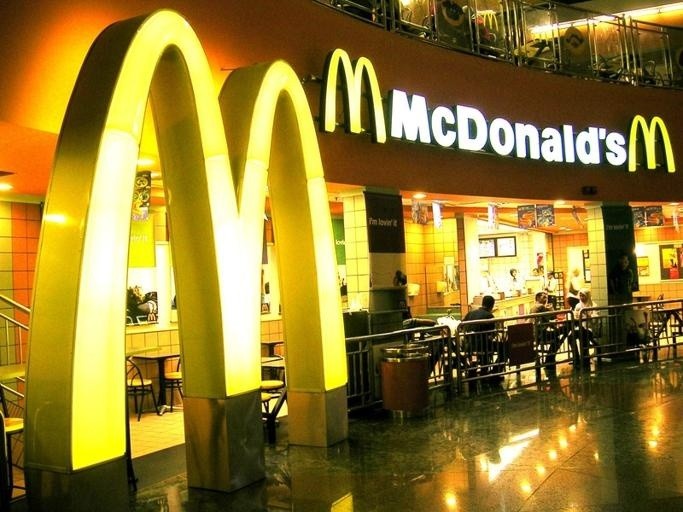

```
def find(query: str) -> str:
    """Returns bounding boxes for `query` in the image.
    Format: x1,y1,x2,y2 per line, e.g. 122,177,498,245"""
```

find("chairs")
1,388,27,512
377,304,504,394
163,358,183,414
649,308,681,338
127,357,159,421
256,340,294,445
351,0,683,91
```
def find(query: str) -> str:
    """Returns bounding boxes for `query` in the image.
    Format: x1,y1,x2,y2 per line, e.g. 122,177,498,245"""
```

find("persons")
482,266,558,310
609,250,634,303
567,287,601,370
457,294,509,384
566,264,584,313
525,290,563,370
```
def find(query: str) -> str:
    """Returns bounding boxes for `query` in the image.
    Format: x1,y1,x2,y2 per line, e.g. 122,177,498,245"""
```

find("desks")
134,347,184,415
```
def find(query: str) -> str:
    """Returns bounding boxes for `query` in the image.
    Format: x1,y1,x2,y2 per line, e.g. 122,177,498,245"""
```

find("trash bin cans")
381,344,431,418
